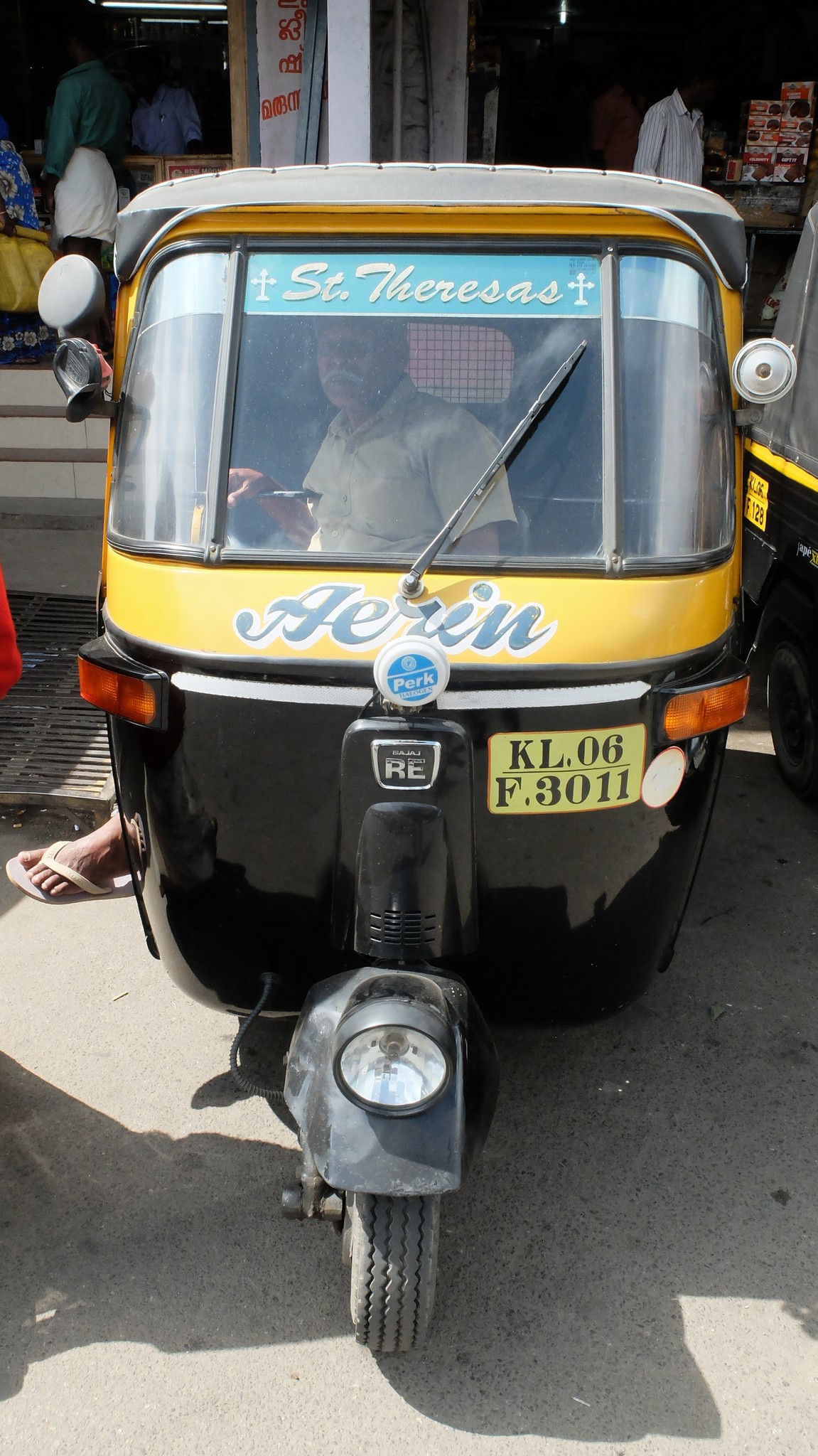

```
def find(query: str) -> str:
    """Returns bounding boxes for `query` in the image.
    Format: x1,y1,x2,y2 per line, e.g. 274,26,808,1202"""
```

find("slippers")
5,841,145,905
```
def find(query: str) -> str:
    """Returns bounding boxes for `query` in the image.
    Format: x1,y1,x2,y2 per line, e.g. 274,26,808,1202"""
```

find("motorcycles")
738,201,818,804
41,159,797,1357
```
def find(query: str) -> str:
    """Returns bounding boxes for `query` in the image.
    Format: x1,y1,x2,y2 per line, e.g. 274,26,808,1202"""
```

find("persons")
128,65,205,159
629,58,721,187
0,118,58,365
0,316,521,904
36,21,126,360
580,56,643,172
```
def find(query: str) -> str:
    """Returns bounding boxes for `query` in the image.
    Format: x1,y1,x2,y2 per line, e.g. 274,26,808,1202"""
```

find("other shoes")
98,344,114,360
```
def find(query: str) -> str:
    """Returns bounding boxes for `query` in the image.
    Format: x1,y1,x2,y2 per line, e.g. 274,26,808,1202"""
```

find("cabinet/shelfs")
707,177,812,336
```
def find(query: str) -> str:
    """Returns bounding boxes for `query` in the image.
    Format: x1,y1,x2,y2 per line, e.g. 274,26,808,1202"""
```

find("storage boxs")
704,79,816,186
